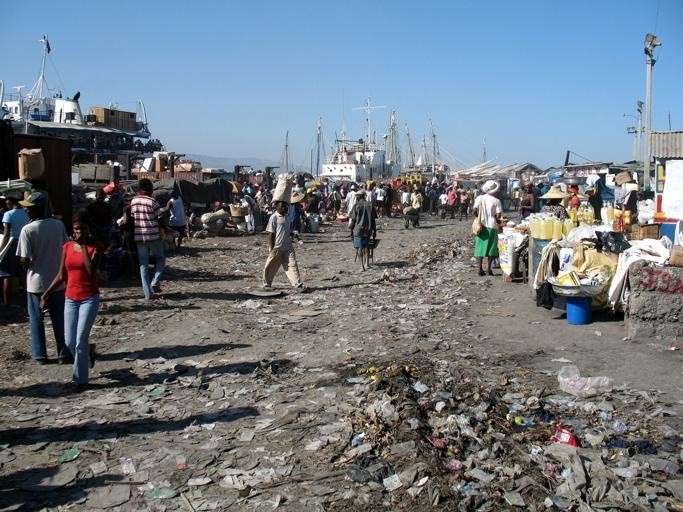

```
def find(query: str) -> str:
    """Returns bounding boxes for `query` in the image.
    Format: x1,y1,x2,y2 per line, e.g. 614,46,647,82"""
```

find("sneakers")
36,359,47,365
151,286,163,293
71,383,90,394
58,356,71,365
88,343,96,369
296,286,307,292
264,285,274,290
478,271,491,275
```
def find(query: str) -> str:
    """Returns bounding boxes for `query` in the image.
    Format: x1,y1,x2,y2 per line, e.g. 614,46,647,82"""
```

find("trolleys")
349,228,380,264
401,212,420,228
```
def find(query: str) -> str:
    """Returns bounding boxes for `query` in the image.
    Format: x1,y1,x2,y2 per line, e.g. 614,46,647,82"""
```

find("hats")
483,180,499,194
18,192,45,208
584,186,593,193
290,192,305,204
567,188,577,194
356,190,365,195
539,185,569,200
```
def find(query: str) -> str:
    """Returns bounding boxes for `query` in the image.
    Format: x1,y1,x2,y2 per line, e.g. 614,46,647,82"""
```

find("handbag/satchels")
472,217,482,235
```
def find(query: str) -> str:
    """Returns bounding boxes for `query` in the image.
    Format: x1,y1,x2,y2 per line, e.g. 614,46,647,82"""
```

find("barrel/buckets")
528,203,595,240
566,296,593,326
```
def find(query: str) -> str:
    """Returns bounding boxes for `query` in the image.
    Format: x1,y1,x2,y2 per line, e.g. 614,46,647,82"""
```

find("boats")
0,33,203,184
321,96,449,186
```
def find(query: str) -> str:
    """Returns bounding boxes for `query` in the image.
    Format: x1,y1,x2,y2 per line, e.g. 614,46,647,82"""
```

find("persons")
341,176,479,229
105,136,164,155
38,211,104,393
469,178,502,276
128,178,173,303
0,189,31,320
13,189,75,365
336,190,376,271
242,176,344,234
260,199,309,294
160,185,187,249
513,176,601,223
